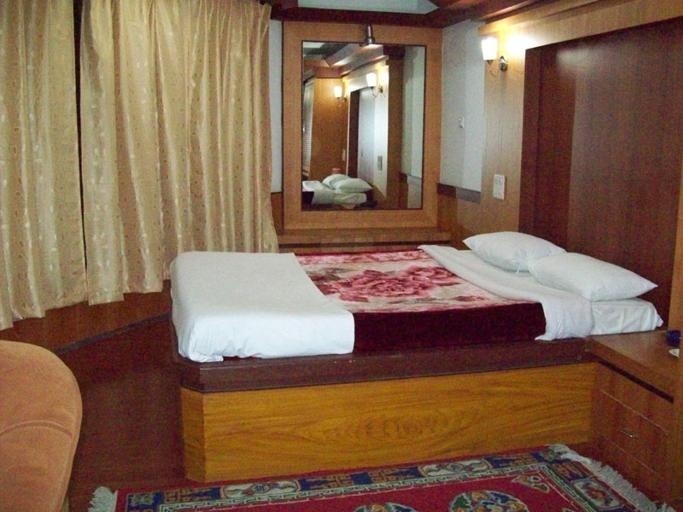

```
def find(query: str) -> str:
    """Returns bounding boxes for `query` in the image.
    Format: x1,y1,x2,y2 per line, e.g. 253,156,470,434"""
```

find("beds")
168,245,660,485
302,179,386,209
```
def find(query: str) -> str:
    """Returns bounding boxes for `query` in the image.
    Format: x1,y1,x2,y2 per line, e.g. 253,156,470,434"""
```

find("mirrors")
281,21,444,229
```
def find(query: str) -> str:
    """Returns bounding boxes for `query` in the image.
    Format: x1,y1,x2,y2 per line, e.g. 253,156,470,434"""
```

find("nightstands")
584,328,683,512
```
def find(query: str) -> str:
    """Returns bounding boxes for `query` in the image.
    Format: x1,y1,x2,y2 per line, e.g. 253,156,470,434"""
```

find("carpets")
83,442,676,511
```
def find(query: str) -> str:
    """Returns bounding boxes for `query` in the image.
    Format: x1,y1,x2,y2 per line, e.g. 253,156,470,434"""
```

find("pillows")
323,173,373,194
462,230,660,302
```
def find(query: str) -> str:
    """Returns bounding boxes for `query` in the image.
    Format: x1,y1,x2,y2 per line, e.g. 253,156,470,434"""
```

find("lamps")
362,24,383,50
363,70,389,98
479,36,509,71
333,85,348,102
